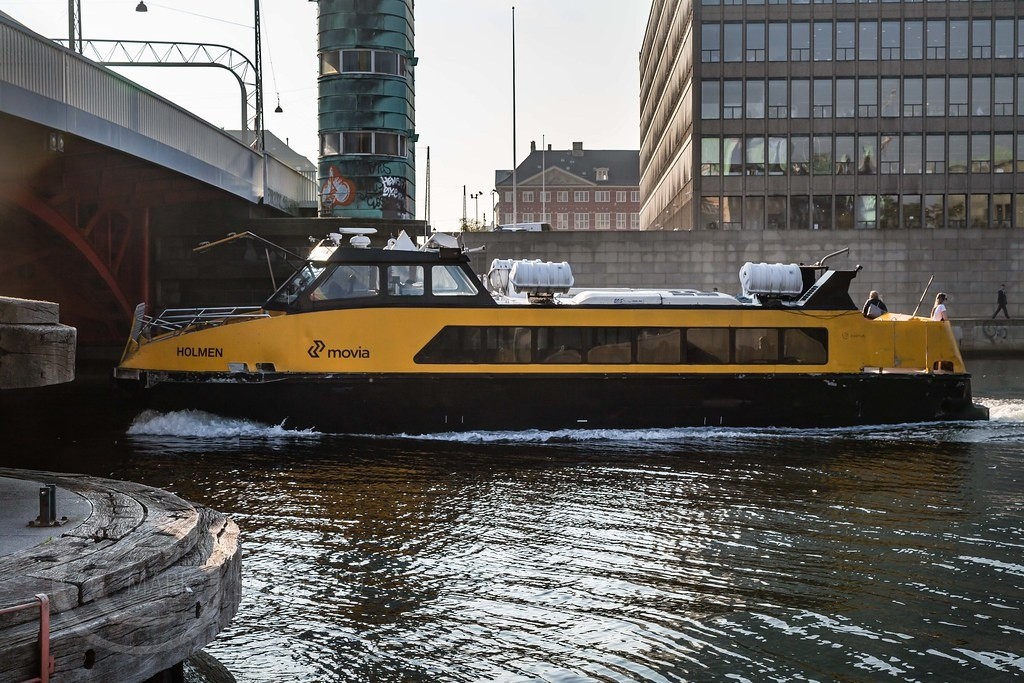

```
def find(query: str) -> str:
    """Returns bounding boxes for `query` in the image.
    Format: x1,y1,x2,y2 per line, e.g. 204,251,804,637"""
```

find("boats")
113,228,990,435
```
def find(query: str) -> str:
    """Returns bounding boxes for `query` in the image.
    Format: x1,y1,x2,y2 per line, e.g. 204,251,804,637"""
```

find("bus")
494,222,554,232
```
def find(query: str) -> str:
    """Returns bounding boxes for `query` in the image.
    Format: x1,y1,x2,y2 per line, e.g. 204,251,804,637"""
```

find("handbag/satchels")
867,302,883,318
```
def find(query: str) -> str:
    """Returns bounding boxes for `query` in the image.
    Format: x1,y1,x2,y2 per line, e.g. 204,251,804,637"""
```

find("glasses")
944,298,947,300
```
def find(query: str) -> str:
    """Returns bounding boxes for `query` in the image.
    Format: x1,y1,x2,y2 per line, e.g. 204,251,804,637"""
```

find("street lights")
471,191,483,228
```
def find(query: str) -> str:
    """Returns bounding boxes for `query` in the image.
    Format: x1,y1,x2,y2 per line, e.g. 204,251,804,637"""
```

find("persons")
930,293,948,321
992,284,1012,319
863,290,888,318
